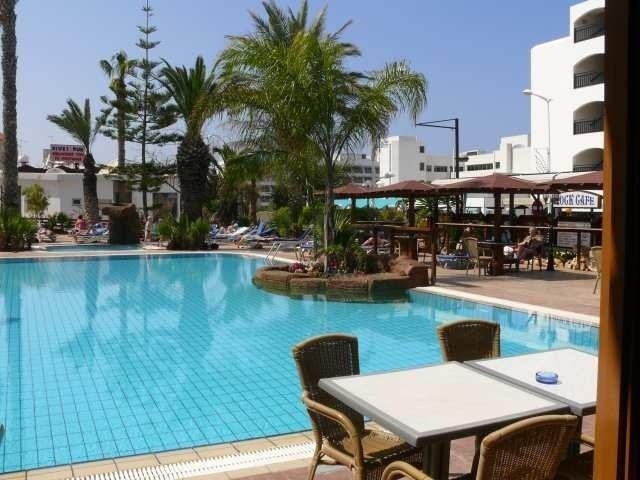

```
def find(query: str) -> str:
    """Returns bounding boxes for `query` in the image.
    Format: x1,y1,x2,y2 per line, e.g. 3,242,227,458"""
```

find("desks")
478,241,517,274
318,361,571,480
463,347,599,455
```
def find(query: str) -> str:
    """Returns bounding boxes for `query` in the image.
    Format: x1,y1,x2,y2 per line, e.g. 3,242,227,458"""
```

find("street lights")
523,89,558,172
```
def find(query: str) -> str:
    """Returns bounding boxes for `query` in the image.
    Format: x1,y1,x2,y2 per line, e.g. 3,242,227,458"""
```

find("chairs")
293,333,423,480
523,235,544,273
71,223,312,250
437,320,500,455
591,246,602,294
381,414,580,480
559,431,595,480
463,237,495,278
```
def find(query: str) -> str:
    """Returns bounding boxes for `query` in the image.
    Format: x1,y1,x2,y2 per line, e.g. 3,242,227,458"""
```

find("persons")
79,222,109,236
517,225,543,259
360,236,390,246
152,217,163,234
219,223,238,234
38,223,55,242
75,214,88,230
144,215,153,242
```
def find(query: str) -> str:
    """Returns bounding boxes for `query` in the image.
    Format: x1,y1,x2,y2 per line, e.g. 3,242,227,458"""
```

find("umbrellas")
535,169,605,191
364,178,435,227
313,182,377,223
430,173,563,226
